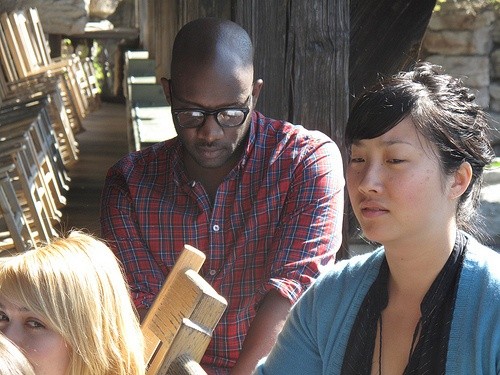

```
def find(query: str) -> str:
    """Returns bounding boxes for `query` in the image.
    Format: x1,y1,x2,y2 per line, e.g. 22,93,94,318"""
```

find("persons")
0,232,145,375
251,61,500,375
101,17,346,375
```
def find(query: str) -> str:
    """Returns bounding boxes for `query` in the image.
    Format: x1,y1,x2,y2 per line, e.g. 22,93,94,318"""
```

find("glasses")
168,79,256,129
